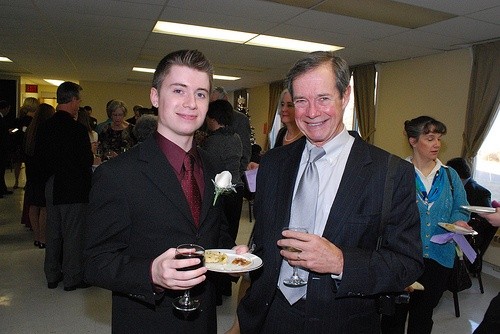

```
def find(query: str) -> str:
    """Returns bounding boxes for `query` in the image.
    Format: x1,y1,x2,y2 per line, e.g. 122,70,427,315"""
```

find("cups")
94,148,103,159
121,148,130,153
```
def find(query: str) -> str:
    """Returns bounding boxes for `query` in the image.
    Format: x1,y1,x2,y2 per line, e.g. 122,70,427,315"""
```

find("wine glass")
105,150,113,161
172,244,205,311
280,227,308,286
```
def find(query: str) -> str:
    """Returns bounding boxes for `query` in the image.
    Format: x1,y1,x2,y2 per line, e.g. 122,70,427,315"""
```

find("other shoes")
39,243,46,248
34,240,40,246
64,281,92,291
46,272,63,288
3,191,13,195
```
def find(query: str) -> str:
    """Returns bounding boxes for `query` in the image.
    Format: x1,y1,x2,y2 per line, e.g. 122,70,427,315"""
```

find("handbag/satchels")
444,167,482,279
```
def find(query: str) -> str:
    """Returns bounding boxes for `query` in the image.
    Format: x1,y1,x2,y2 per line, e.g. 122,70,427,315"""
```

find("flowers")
212,170,236,205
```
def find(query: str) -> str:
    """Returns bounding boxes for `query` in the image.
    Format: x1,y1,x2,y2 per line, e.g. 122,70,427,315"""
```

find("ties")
181,153,202,232
278,147,325,306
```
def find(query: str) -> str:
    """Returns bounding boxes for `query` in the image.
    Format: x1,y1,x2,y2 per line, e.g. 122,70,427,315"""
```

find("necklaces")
285,130,301,141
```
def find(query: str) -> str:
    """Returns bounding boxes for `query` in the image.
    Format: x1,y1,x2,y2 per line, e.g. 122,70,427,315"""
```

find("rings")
298,252,301,261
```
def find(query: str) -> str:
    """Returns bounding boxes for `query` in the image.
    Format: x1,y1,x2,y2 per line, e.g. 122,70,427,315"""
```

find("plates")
412,282,424,290
461,206,496,214
196,249,264,273
438,223,478,235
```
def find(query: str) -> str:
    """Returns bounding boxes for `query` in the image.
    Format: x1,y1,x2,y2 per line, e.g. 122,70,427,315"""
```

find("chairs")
452,216,500,317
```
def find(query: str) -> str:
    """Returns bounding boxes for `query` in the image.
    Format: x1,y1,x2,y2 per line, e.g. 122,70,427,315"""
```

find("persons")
79,50,247,334
237,50,424,334
473,206,500,334
274,88,304,148
446,157,493,273
0,81,264,291
380,115,472,334
